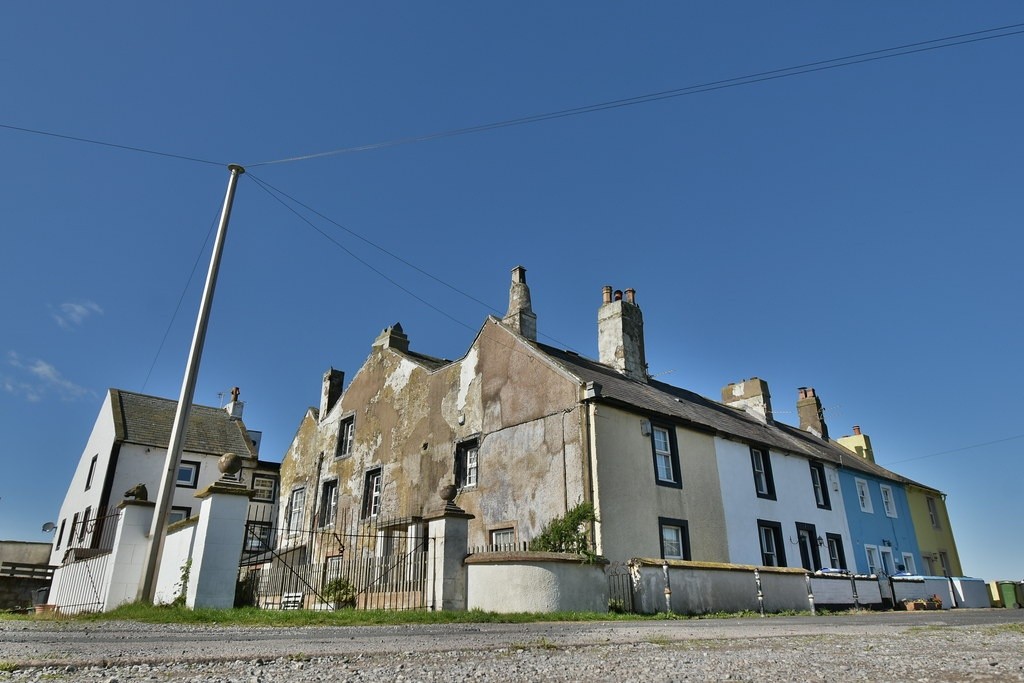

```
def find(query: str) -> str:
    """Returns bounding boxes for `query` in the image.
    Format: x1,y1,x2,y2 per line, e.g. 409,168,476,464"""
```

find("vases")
311,601,353,611
904,602,942,611
27,607,36,615
35,603,57,619
